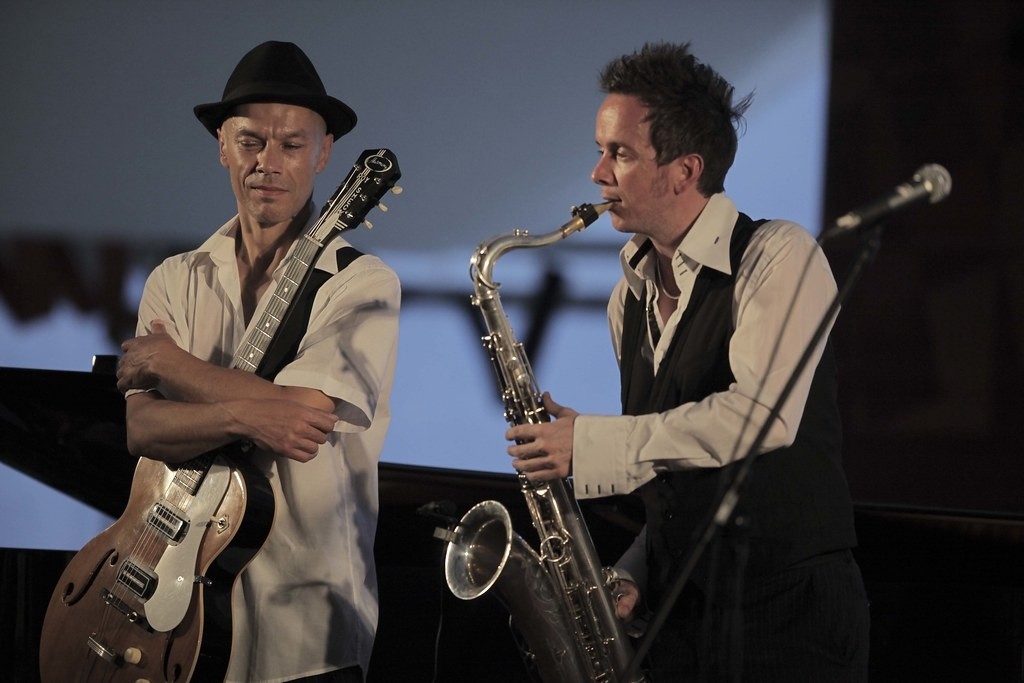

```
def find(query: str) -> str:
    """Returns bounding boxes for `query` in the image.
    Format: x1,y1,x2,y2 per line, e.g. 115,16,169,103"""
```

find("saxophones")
445,201,634,683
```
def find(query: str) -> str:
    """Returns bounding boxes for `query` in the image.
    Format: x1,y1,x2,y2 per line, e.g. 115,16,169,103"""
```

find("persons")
117,40,402,683
504,42,870,683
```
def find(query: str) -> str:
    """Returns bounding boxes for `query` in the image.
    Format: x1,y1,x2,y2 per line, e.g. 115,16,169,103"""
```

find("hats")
193,40,357,143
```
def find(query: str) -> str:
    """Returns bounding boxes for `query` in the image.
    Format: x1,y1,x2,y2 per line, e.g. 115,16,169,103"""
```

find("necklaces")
656,257,681,300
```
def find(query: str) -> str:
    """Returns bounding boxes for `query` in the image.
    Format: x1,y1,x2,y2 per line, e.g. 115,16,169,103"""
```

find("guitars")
38,148,402,683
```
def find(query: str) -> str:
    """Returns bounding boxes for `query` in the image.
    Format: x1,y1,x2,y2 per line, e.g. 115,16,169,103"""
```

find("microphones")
816,163,952,242
